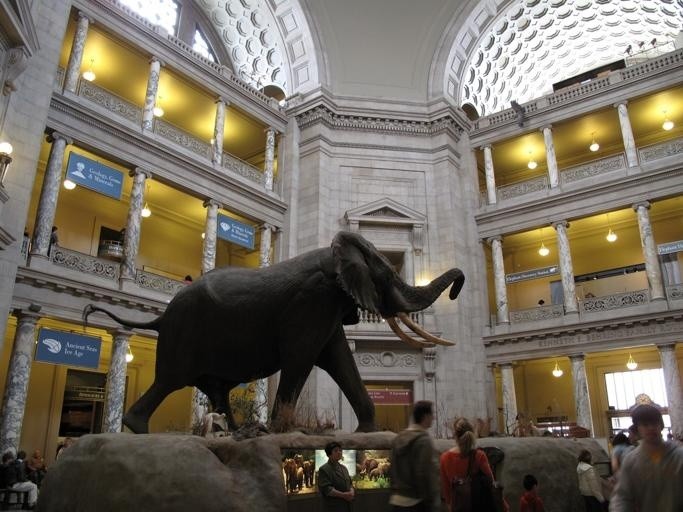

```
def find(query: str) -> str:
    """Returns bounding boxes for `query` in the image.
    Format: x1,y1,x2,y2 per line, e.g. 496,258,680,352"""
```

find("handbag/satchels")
451,449,504,512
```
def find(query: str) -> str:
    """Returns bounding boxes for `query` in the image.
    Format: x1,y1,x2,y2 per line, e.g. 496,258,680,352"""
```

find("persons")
517,473,547,511
27,448,47,485
316,441,356,511
583,291,596,298
447,418,468,439
0,450,13,487
182,274,193,287
536,298,546,307
45,223,60,258
439,417,509,511
55,436,73,461
387,399,436,511
4,449,39,508
575,402,683,511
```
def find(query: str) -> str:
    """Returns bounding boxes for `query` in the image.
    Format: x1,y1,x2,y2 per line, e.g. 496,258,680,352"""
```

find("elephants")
355,455,391,483
283,454,315,494
81,229,465,435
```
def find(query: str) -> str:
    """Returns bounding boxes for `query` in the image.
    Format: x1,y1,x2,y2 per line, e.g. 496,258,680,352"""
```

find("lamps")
140,181,153,217
83,59,96,82
525,148,538,170
151,92,167,118
585,129,603,152
548,355,565,378
603,212,617,244
535,226,551,258
623,349,638,371
658,108,674,130
